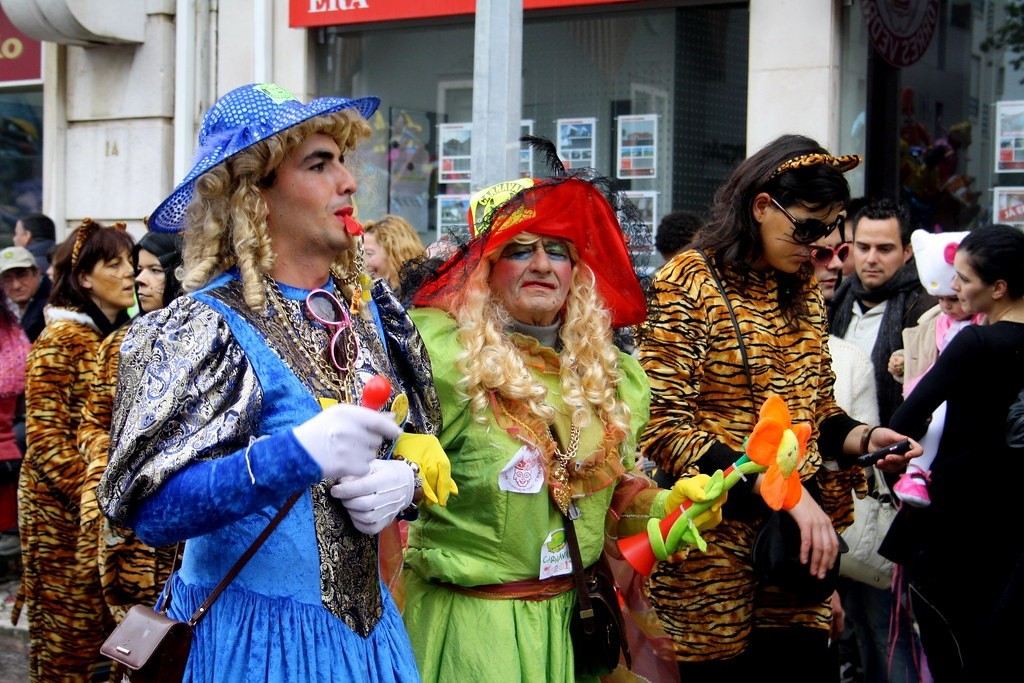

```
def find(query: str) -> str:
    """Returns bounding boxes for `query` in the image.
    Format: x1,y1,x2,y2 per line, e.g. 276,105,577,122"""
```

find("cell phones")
858,441,914,468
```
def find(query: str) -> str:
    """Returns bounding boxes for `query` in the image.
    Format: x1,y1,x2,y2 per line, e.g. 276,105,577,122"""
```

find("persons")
0,213,60,537
837,193,1024,683
79,229,188,683
394,177,650,683
98,83,413,683
16,213,136,683
632,133,925,683
360,215,424,294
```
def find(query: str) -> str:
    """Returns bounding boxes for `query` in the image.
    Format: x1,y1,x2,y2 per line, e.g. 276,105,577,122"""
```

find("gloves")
331,461,415,535
295,402,404,478
665,470,728,531
395,433,460,506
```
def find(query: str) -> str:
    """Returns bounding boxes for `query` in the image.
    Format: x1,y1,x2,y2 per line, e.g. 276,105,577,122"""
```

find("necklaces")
505,402,585,515
253,267,357,402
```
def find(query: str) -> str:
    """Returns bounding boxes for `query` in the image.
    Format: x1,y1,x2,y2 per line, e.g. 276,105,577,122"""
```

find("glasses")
771,198,837,245
307,289,358,370
811,243,850,269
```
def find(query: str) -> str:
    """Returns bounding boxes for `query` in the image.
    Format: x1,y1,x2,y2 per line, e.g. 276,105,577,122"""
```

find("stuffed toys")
647,393,812,563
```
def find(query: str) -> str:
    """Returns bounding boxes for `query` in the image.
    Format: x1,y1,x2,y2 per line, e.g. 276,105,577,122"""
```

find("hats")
401,135,648,331
911,228,971,296
149,84,381,236
0,246,39,274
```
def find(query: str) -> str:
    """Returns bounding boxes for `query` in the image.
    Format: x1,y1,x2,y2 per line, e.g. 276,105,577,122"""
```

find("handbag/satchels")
568,575,633,677
838,495,900,590
750,509,849,609
100,604,193,683
864,564,933,683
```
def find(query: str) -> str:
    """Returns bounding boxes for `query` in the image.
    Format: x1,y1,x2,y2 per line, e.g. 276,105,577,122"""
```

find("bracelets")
859,424,880,456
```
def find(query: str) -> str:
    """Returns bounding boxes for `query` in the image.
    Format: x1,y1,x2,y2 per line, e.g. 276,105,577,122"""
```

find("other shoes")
894,469,934,507
0,532,20,555
